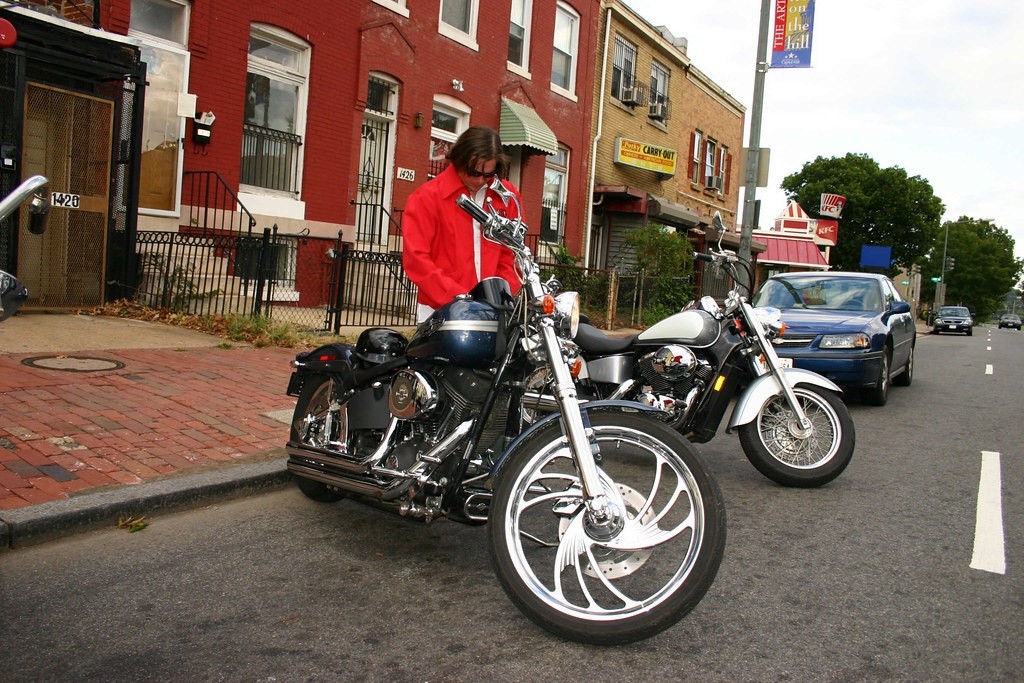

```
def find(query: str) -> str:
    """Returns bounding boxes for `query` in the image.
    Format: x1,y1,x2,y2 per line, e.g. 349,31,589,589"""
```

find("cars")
743,270,918,408
931,306,976,337
998,313,1024,331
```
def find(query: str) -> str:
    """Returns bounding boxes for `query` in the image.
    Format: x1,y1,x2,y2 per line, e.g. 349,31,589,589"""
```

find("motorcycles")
278,193,729,648
508,209,856,490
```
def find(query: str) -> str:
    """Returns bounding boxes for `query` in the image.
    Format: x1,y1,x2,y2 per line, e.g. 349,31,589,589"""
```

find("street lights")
938,218,995,306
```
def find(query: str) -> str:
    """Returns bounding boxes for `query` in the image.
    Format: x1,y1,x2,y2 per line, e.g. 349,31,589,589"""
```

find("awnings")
499,97,558,157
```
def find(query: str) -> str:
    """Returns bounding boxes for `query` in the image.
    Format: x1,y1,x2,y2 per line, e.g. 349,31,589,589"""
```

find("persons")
404,125,527,471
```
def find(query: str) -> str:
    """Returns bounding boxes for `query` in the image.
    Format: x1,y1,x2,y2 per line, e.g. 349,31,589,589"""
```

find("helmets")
353,328,408,364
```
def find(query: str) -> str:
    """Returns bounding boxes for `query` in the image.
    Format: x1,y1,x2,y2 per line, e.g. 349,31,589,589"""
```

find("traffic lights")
946,256,955,272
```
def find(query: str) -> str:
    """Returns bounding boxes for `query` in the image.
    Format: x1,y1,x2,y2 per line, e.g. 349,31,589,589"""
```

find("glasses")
467,167,496,178
